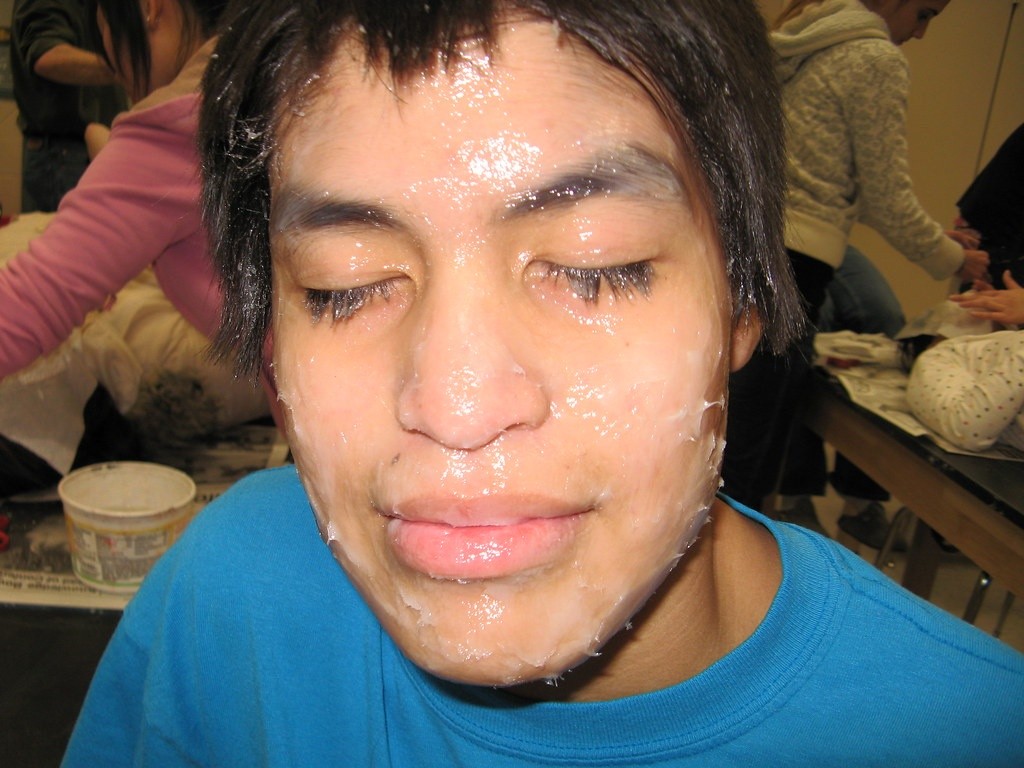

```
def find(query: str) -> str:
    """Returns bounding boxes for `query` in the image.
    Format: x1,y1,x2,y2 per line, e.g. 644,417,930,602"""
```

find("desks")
805,362,1024,642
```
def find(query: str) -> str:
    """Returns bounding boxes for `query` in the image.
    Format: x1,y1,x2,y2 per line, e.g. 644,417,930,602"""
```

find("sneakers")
772,498,827,540
836,502,906,551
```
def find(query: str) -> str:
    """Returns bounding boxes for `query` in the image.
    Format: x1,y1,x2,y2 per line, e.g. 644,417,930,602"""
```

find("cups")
58,460,197,593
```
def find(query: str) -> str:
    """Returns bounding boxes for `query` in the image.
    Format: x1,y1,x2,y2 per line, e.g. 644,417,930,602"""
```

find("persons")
715,0,1024,558
59,0,1024,768
0,0,294,463
